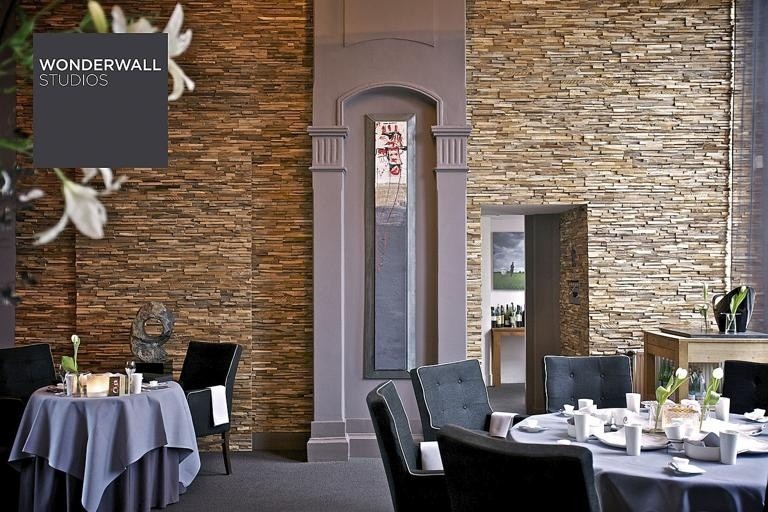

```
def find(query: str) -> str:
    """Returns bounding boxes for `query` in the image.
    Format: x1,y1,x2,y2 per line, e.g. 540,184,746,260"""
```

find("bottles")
491,302,525,328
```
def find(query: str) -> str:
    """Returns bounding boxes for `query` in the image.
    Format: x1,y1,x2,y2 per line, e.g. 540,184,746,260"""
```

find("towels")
420,442,443,471
206,384,229,427
488,411,519,439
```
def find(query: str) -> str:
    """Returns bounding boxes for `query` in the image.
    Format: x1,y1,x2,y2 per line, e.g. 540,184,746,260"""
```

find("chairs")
411,360,525,445
180,341,244,475
437,425,600,512
0,343,57,456
364,379,450,510
543,353,633,411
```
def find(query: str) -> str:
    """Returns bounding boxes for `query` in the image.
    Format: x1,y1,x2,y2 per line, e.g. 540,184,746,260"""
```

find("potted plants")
719,288,749,334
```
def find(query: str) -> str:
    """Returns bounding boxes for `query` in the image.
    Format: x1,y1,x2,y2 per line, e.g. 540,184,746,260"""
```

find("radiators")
617,349,723,401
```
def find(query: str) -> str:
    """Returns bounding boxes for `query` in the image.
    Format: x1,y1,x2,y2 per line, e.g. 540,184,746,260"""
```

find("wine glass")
58,364,71,397
125,361,136,381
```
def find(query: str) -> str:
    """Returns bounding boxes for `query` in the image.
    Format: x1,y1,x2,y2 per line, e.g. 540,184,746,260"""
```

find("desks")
491,328,525,386
644,328,768,409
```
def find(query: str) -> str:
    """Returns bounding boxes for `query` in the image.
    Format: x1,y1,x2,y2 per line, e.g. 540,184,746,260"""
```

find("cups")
150,381,158,387
132,373,142,395
527,393,766,465
66,374,77,395
